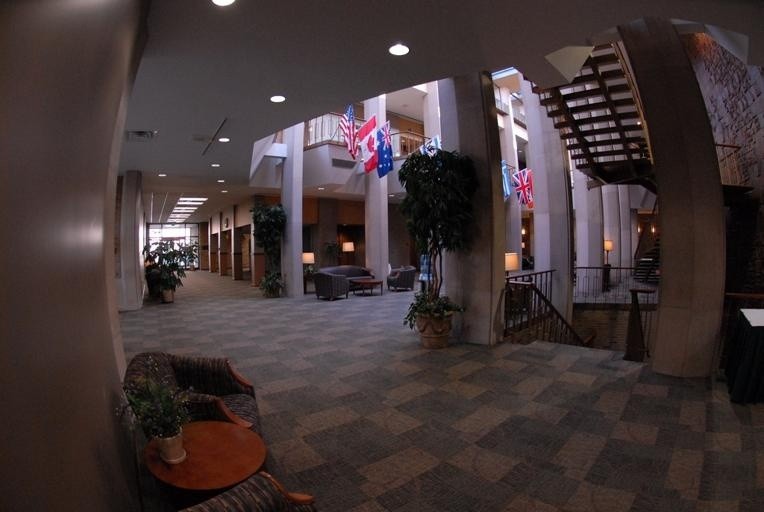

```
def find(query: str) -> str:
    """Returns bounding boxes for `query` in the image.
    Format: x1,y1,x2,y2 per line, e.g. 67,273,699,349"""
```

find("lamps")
504,252,519,277
342,241,356,265
602,239,614,266
302,252,315,265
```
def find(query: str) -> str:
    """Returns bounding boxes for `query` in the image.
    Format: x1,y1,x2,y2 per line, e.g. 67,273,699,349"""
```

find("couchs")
320,265,374,296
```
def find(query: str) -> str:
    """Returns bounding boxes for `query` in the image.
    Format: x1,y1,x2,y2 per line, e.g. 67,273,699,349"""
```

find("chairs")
171,469,317,512
313,271,349,303
118,345,267,438
386,266,416,292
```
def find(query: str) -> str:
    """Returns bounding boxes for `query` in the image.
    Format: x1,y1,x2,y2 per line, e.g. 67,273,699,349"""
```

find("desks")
137,418,269,490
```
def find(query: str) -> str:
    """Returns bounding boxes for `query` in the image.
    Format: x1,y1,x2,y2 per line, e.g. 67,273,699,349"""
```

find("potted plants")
394,143,482,348
243,201,289,299
138,237,200,305
110,350,193,465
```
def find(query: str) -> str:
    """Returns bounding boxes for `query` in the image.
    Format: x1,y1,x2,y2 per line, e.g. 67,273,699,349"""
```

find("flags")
375,122,394,179
513,168,535,206
502,160,513,202
419,135,440,158
355,113,377,174
338,103,359,161
